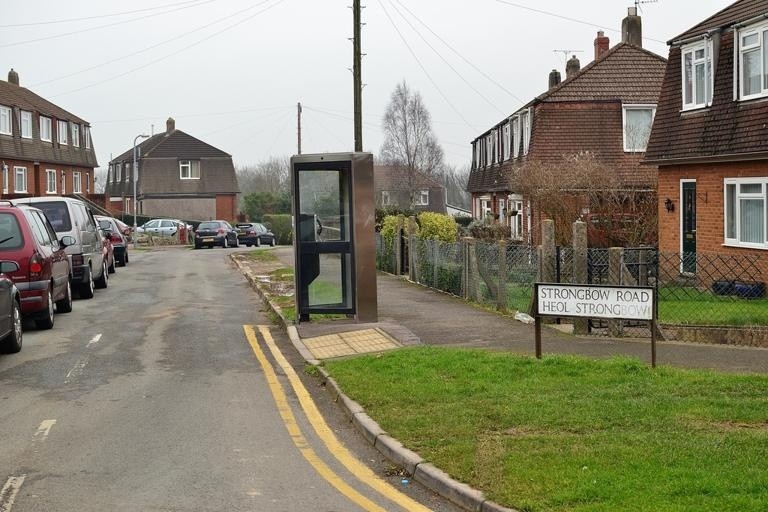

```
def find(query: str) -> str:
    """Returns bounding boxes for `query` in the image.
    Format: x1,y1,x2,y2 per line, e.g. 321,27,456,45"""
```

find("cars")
195,220,240,248
1,196,132,354
581,212,658,248
137,219,193,237
234,223,276,247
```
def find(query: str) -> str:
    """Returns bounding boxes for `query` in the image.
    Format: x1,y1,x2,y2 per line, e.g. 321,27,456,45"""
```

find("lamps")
503,208,506,215
665,198,674,213
61,171,66,177
93,177,97,182
1,161,5,169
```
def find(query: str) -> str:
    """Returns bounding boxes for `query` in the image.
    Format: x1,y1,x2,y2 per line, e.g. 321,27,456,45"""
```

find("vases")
712,280,736,295
734,280,765,299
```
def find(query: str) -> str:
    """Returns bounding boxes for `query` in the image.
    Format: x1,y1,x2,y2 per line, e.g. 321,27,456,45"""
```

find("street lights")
133,134,149,249
